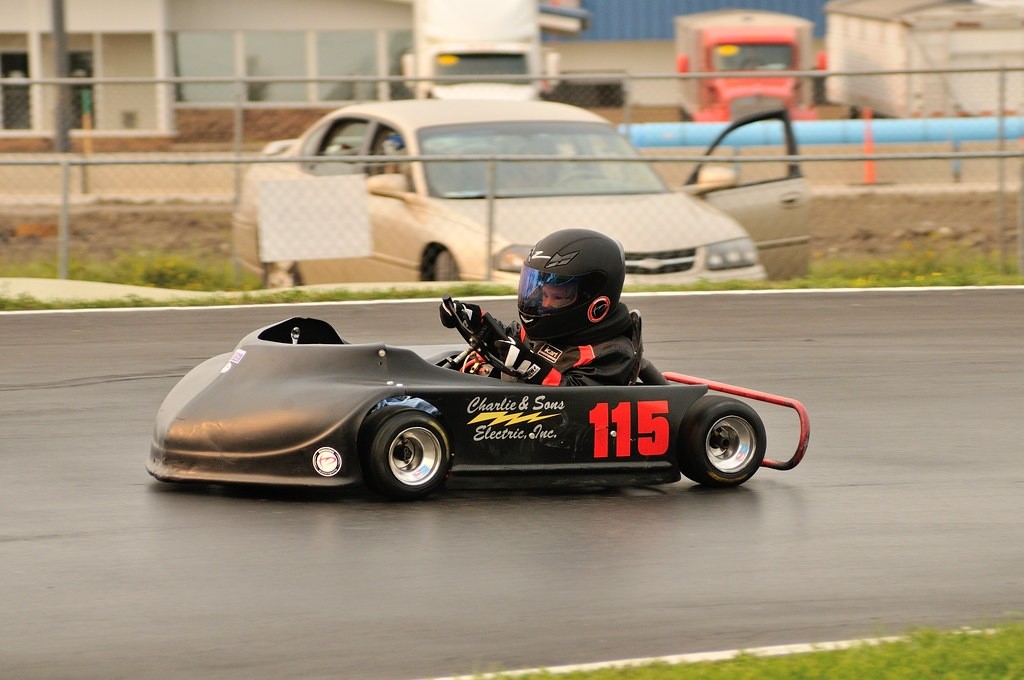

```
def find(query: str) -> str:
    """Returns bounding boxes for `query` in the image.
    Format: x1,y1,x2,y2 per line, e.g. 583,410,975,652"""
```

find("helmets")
517,229,625,337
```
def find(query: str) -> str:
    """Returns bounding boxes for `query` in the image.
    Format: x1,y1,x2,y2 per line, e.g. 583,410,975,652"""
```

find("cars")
230,98,813,291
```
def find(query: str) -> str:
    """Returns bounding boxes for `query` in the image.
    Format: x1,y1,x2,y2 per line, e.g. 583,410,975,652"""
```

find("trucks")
401,0,562,103
675,8,828,121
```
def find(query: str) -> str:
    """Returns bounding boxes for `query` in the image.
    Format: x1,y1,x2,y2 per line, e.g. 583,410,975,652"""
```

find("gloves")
439,300,481,330
494,333,554,384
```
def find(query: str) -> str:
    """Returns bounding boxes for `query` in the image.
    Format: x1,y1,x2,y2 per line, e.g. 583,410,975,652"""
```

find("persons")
439,228,636,387
